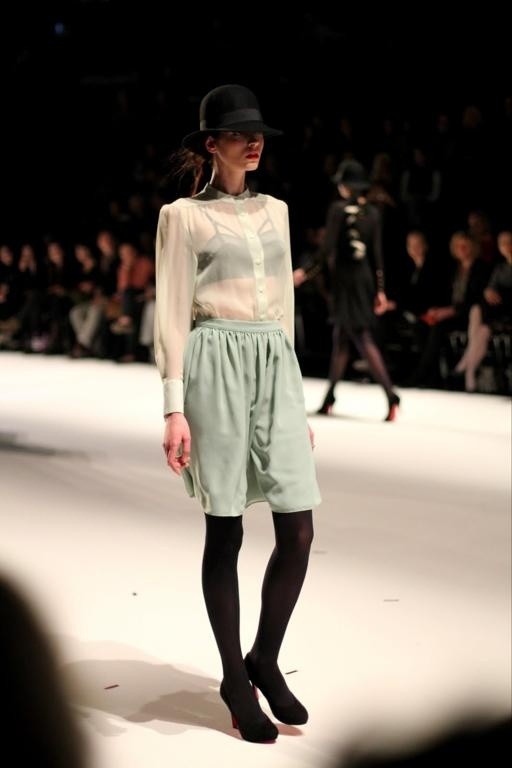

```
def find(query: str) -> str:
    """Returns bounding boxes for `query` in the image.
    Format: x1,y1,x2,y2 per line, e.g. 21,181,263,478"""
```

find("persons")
293,158,401,422
154,86,322,746
297,226,512,397
1,233,156,366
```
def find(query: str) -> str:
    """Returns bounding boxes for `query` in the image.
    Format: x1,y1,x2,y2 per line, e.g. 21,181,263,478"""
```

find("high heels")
219,674,278,743
242,650,312,725
383,394,400,423
316,392,335,416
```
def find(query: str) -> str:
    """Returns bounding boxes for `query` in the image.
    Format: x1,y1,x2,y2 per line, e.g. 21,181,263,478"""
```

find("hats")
328,159,373,193
182,83,285,160
